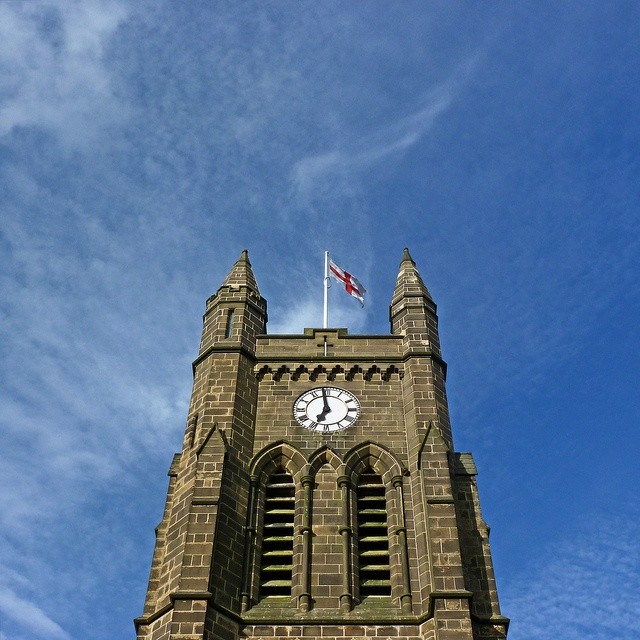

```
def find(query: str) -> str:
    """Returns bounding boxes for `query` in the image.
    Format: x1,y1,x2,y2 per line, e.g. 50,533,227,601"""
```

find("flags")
323,251,367,307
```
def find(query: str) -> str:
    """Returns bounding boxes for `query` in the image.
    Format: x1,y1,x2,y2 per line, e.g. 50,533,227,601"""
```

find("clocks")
291,384,363,435
